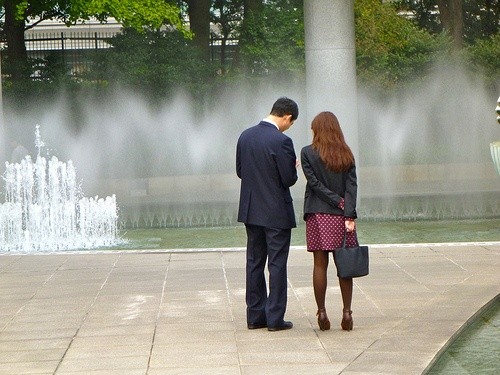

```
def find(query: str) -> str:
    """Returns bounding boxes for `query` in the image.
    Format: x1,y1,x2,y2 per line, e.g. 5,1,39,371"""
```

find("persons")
11,137,29,163
236,98,300,331
300,112,360,331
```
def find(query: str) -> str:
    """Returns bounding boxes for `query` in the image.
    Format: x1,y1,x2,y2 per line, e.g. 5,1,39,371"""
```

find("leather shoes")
248,322,267,329
268,321,293,331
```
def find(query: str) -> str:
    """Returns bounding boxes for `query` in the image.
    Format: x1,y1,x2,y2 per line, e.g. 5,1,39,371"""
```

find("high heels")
341,311,353,332
316,310,330,331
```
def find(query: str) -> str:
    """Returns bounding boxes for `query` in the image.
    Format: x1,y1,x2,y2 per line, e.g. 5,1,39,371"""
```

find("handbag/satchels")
334,228,369,279
